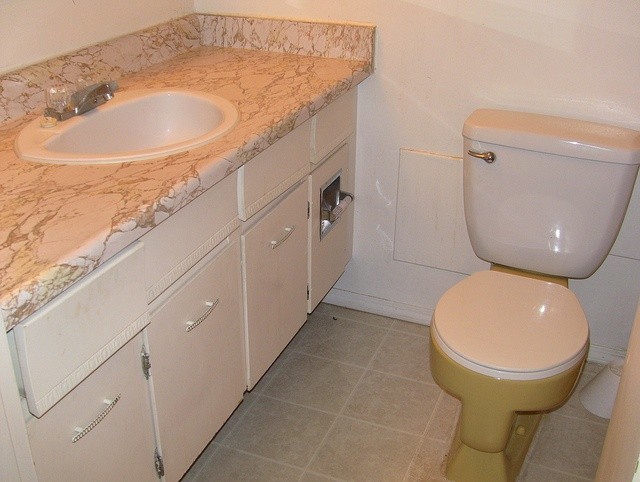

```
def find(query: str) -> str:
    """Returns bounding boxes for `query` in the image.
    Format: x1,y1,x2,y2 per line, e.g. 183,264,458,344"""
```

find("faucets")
68,80,118,115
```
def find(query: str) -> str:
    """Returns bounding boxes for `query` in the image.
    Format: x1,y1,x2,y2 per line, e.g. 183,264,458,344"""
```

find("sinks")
40,89,228,156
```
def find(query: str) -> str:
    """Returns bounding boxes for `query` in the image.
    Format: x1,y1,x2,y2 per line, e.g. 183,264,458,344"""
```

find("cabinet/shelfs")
308,84,359,314
7,238,166,481
137,167,247,482
236,116,315,394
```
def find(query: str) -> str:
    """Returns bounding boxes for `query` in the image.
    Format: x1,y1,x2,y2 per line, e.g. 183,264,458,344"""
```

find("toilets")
428,106,640,482
